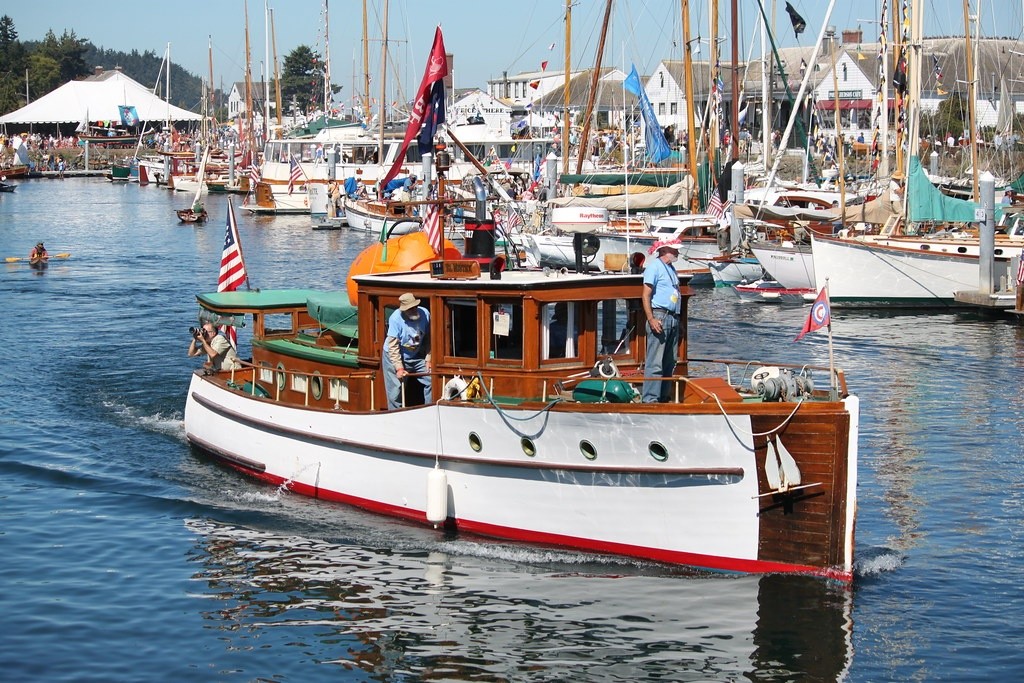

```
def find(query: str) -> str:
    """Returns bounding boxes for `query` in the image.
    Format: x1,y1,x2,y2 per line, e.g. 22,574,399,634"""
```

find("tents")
0,70,221,124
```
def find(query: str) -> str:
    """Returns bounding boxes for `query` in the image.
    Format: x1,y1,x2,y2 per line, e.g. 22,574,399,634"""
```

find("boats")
184,23,859,583
178,144,210,223
29,257,48,270
0,167,30,192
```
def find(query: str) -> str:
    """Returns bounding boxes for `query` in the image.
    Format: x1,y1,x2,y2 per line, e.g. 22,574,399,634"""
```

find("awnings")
816,99,903,110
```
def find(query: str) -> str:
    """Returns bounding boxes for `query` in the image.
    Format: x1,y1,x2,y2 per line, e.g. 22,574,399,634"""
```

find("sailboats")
106,0,1024,326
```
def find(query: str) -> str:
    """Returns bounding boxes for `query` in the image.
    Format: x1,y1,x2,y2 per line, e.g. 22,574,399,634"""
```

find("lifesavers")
443,378,468,400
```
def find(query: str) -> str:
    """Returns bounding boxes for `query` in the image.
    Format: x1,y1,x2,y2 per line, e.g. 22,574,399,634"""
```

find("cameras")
189,326,207,338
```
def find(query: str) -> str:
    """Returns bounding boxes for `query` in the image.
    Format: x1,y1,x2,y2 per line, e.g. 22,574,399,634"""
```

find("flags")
380,29,447,194
251,155,261,183
785,1,806,36
288,156,302,194
216,204,245,351
424,186,441,253
707,188,731,219
621,65,670,164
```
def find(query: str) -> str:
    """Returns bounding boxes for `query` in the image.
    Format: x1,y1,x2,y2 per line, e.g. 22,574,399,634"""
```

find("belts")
652,308,678,317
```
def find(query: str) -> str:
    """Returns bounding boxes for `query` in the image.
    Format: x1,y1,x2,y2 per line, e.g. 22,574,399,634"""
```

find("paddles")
5,253,70,262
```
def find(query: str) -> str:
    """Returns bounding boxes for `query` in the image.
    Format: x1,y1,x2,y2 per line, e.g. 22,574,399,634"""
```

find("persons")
194,200,203,213
188,323,241,370
29,241,49,261
642,235,685,403
2,131,98,171
328,148,379,217
918,131,1020,153
890,170,905,235
144,125,239,153
383,293,432,410
236,163,243,186
664,124,782,156
402,175,418,215
1003,186,1014,204
549,128,642,156
807,132,865,157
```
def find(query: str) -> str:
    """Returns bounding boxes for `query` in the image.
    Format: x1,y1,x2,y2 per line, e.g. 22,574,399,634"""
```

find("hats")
398,292,421,310
654,234,684,251
